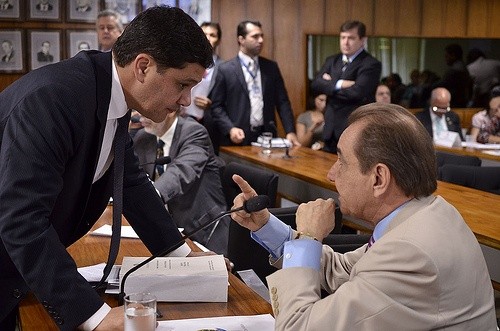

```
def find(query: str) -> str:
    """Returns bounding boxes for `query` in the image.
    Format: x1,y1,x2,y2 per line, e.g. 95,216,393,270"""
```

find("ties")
95,108,132,290
248,59,263,122
363,235,375,253
435,117,445,136
157,140,166,176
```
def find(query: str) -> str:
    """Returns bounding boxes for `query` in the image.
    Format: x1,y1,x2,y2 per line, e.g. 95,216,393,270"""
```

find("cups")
261,133,272,155
123,292,157,331
466,135,475,152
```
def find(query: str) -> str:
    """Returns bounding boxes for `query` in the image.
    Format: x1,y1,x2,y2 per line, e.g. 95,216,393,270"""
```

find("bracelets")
294,232,318,241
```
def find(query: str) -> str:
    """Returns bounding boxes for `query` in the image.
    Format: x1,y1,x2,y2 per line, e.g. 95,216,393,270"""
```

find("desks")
433,142,500,162
17,202,276,331
220,142,500,291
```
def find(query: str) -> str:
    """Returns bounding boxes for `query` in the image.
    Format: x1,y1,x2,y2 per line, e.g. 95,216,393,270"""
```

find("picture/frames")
0,0,212,76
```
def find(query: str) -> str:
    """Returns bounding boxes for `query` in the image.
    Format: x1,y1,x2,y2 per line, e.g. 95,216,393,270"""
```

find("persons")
210,20,302,147
77,41,90,51
232,102,498,331
133,107,231,258
310,20,382,154
1,40,18,65
96,12,123,52
465,50,500,107
375,69,440,109
181,21,223,157
0,5,216,331
416,86,465,143
295,89,327,149
0,0,13,10
440,43,474,109
36,0,53,11
75,1,204,17
37,39,53,62
470,89,500,144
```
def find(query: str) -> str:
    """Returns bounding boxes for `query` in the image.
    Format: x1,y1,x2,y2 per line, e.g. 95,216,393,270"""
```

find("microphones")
270,120,293,159
119,195,270,307
141,155,172,167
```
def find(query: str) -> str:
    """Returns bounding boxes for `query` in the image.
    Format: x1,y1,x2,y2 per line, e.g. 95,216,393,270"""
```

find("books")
119,254,227,302
